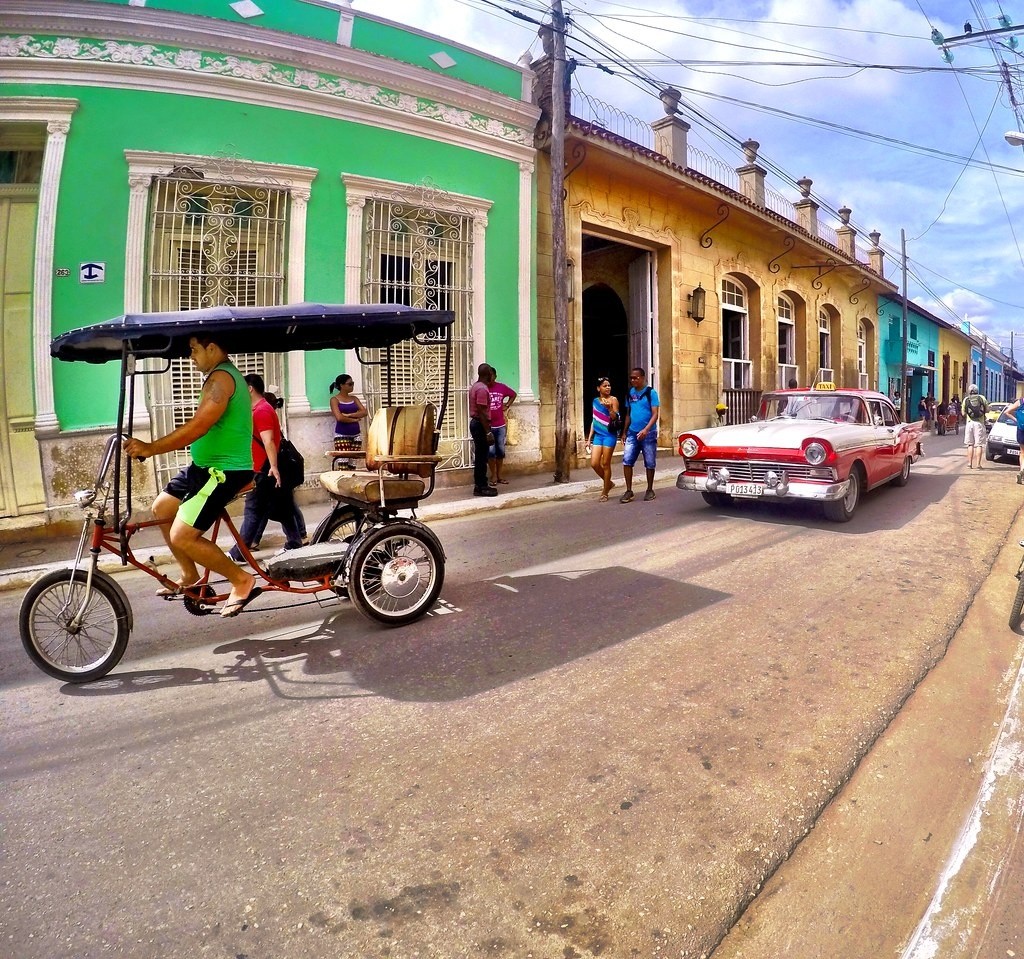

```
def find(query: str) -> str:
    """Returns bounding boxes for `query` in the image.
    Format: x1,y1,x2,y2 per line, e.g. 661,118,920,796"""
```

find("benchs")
319,401,435,510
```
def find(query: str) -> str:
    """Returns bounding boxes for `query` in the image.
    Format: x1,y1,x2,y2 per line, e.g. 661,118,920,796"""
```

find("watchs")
505,404,509,408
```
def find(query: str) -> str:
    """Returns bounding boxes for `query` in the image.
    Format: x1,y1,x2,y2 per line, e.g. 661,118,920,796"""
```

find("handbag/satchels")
262,436,304,487
608,411,622,437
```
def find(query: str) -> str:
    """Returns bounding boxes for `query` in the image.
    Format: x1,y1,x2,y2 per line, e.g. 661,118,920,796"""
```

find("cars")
676,382,925,522
985,403,1021,465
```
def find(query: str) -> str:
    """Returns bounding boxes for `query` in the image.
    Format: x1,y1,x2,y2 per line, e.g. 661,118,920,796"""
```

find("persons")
876,391,901,418
225,373,308,564
584,378,619,502
123,330,255,616
962,385,990,470
777,379,797,415
469,364,498,496
620,368,660,502
1005,398,1024,483
919,392,962,431
330,374,368,471
489,368,517,486
707,403,728,427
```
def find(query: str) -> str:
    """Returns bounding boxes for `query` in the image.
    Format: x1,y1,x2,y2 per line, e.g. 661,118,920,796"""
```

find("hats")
968,384,978,394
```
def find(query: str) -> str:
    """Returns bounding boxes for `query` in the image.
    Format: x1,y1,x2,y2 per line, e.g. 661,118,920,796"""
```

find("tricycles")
18,304,455,682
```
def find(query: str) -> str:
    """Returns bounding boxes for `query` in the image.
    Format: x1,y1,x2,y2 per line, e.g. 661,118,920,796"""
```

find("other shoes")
603,483,615,495
473,486,498,497
600,495,608,502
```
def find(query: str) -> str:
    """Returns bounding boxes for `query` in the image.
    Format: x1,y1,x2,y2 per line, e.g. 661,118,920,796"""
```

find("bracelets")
487,431,491,435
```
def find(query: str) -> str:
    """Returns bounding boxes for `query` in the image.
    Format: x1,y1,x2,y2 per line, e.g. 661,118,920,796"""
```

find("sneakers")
1016,473,1023,485
644,490,656,501
966,465,972,469
977,466,983,471
620,491,635,502
222,551,249,564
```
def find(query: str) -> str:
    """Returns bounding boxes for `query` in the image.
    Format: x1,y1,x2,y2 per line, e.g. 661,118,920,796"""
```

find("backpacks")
966,395,984,418
1016,398,1024,430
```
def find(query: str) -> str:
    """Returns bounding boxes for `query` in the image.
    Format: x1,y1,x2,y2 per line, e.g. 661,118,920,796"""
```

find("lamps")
688,281,706,327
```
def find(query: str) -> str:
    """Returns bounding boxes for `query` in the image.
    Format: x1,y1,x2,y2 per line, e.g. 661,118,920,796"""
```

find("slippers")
221,587,262,618
156,576,197,595
490,480,497,485
498,478,509,484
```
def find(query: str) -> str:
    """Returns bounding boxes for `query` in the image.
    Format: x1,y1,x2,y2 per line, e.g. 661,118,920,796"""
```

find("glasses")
344,382,354,386
598,377,608,381
630,375,640,380
